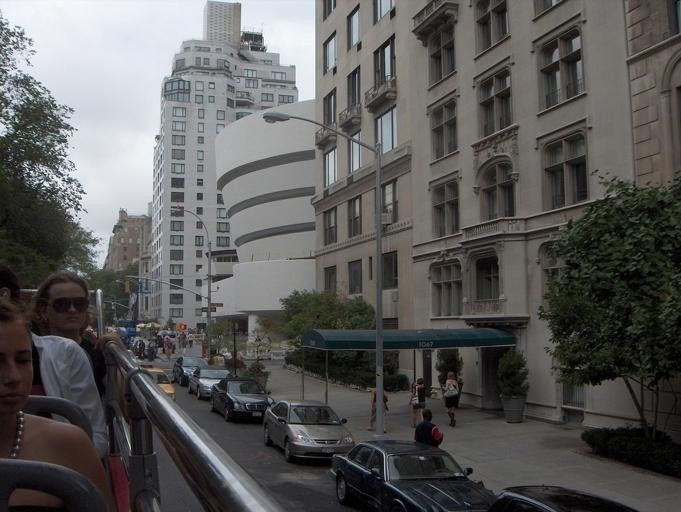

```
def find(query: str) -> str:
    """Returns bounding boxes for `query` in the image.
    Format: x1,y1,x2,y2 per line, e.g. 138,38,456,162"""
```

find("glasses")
45,296,93,314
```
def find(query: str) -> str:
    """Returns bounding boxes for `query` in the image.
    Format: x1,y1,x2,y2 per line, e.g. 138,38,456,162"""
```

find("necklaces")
9,410,26,459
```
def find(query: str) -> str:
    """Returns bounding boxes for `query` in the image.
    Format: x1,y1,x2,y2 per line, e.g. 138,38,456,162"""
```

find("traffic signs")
202,307,216,312
211,303,223,307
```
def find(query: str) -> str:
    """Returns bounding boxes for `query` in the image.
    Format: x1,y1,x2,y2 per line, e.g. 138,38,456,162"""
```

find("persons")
415,408,446,471
409,377,426,427
0,298,115,511
29,270,125,402
126,322,272,369
444,371,461,426
368,387,389,433
0,263,112,456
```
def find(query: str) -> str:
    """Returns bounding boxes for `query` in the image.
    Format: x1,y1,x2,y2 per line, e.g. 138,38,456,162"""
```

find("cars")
492,485,639,511
142,364,176,401
174,356,208,386
211,378,275,422
188,367,231,399
331,440,496,511
265,399,355,463
128,336,150,356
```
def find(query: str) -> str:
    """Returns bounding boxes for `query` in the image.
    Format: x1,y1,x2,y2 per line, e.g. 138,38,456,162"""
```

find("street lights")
264,112,385,436
126,208,213,364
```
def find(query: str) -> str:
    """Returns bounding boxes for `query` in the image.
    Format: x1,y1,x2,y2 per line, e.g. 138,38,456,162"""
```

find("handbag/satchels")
443,383,458,397
409,386,419,406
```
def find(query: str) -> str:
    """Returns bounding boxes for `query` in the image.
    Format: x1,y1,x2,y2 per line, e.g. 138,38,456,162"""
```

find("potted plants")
435,348,465,407
496,348,531,423
